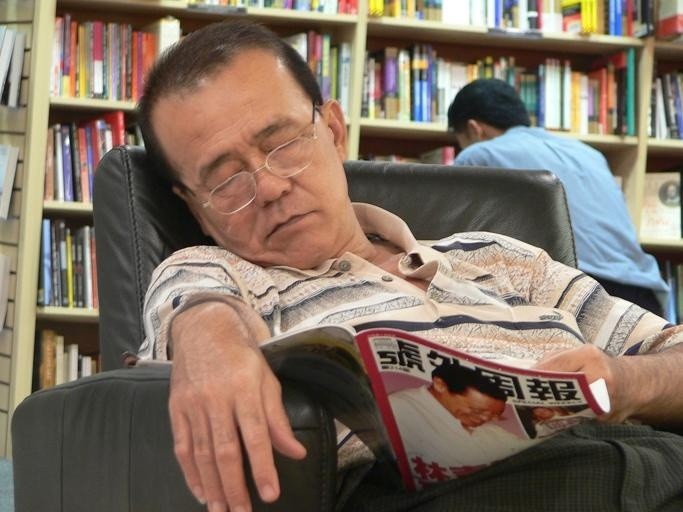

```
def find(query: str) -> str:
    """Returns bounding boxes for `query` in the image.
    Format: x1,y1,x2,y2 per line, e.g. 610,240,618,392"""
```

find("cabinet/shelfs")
0,3,681,460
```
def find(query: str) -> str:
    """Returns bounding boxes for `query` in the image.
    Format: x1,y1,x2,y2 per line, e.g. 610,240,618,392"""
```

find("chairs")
7,142,583,511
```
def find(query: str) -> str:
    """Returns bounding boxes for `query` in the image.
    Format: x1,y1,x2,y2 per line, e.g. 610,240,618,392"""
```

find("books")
37,14,157,310
33,329,97,391
134,324,611,493
203,0,683,165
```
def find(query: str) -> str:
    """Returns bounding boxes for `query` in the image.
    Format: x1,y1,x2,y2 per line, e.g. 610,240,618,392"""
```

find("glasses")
181,97,316,215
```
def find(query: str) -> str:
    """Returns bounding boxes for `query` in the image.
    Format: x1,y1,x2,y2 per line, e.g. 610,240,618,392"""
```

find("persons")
123,17,683,512
533,406,574,438
388,364,541,492
